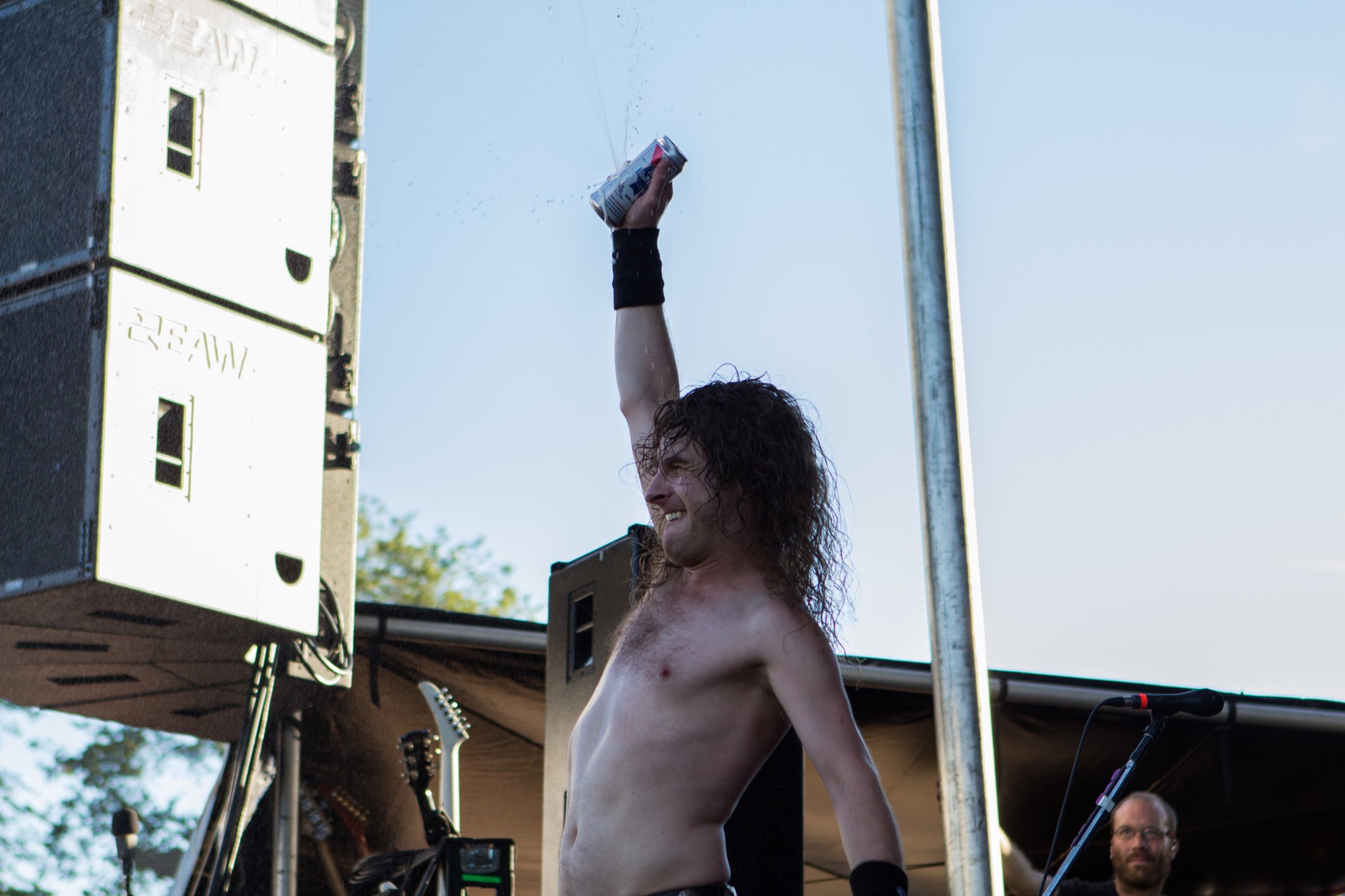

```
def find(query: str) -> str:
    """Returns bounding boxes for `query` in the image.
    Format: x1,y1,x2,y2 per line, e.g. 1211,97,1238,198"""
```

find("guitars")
395,727,440,846
418,680,472,896
328,787,370,861
299,784,350,896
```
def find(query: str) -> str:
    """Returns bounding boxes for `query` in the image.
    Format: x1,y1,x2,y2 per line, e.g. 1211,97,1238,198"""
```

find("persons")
1006,792,1179,896
559,159,908,896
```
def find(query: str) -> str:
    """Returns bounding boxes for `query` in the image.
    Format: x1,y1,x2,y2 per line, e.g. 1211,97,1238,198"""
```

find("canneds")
589,134,688,228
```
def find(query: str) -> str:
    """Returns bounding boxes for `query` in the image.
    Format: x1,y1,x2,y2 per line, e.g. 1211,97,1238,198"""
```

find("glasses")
1114,824,1169,841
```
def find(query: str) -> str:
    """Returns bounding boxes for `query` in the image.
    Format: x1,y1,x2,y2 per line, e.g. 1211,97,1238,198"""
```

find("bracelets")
849,860,908,896
611,228,665,310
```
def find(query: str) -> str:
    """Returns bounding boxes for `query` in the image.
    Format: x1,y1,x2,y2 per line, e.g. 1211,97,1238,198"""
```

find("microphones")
1109,689,1225,717
111,809,139,876
